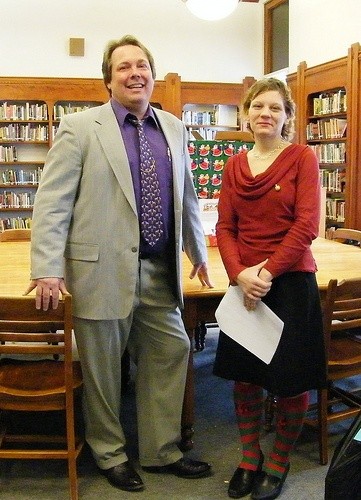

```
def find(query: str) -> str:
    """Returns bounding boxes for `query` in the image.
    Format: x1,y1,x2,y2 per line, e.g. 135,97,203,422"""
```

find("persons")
22,37,212,494
214,77,327,500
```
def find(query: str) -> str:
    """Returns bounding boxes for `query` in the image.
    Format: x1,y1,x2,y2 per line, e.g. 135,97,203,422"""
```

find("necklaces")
253,138,286,160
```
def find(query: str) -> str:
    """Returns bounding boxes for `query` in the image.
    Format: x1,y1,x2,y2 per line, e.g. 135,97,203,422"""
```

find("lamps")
185,0,239,23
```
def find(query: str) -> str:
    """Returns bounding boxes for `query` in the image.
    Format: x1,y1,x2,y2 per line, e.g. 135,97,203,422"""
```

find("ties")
126,116,163,246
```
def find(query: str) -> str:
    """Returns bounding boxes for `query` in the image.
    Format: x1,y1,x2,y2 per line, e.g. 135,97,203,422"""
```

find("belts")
139,250,176,262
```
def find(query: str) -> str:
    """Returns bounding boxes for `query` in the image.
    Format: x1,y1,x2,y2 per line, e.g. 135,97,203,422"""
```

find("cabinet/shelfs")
0,45,361,246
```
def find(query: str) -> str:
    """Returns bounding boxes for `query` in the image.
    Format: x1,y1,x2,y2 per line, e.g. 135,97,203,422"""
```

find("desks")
0,238,361,451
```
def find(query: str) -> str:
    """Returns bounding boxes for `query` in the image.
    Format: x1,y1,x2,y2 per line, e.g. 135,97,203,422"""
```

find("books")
307,88,349,224
0,103,217,234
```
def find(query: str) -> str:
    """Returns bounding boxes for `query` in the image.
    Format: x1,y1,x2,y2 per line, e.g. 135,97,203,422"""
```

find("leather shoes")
250,459,291,500
103,463,144,490
228,451,265,498
142,455,211,477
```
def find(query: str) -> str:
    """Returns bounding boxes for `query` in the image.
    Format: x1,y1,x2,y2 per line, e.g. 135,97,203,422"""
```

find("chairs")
0,293,87,500
264,280,361,468
